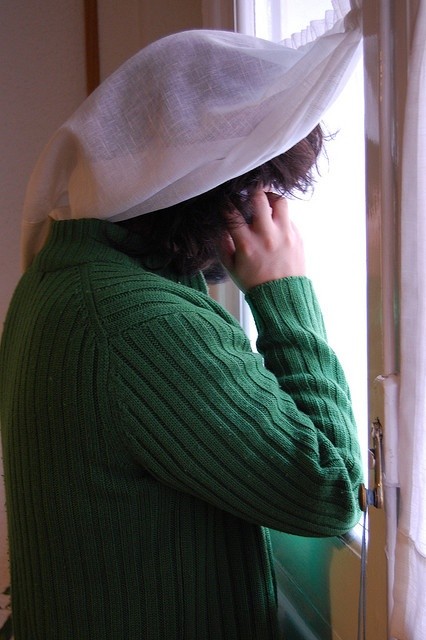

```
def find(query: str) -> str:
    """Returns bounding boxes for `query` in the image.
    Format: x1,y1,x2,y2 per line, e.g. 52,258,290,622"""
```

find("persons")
2,30,363,639
